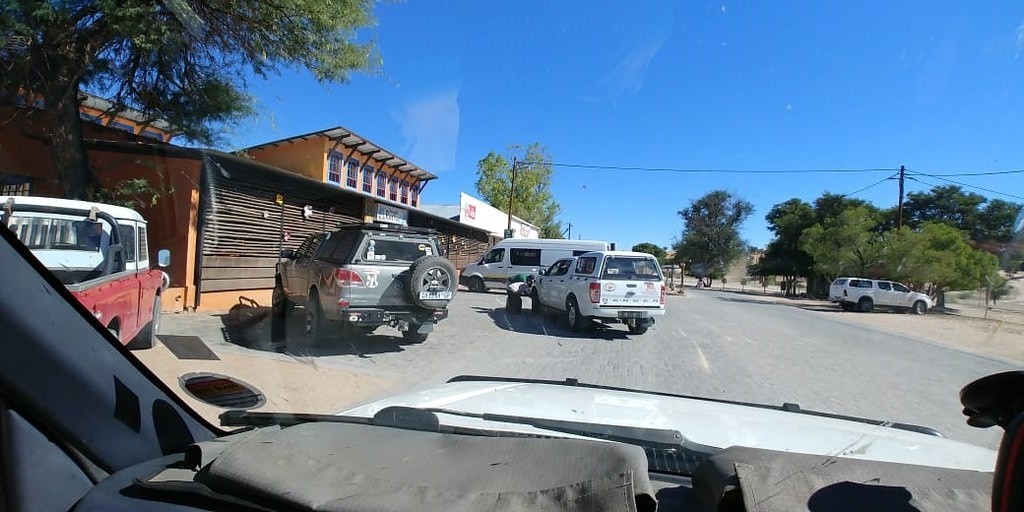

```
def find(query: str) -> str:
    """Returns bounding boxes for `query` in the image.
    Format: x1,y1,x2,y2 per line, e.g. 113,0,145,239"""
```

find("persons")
505,273,535,314
507,281,531,315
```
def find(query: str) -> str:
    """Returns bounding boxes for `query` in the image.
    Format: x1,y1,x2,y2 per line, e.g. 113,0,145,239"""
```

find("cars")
660,266,682,279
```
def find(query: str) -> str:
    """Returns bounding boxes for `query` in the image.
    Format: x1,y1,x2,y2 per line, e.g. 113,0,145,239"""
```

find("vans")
459,238,612,293
530,250,665,335
827,277,933,315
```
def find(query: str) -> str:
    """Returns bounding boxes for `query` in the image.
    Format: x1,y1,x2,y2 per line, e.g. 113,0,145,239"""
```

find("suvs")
272,223,459,344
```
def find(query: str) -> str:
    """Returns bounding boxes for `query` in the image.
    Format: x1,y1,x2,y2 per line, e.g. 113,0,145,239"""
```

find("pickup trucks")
0,196,170,348
